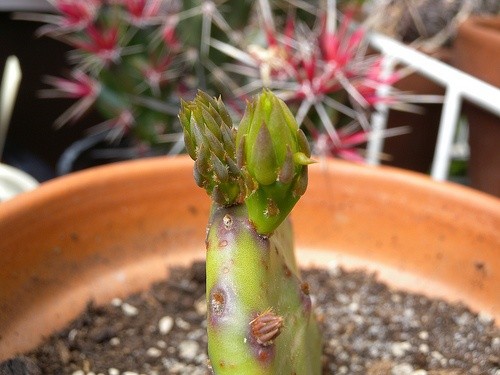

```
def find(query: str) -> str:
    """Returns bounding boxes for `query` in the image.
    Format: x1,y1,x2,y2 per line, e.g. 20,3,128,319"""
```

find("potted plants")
0,88,500,375
